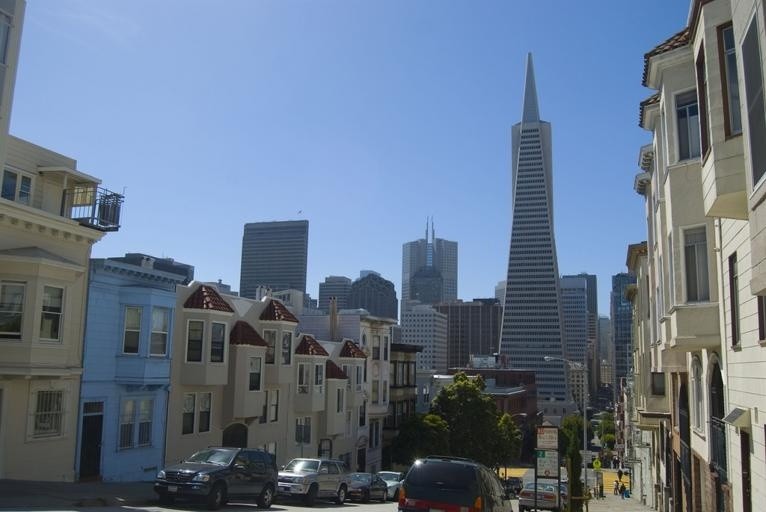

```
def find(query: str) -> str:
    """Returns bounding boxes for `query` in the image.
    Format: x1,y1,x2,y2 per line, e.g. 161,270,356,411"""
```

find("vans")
397,454,516,512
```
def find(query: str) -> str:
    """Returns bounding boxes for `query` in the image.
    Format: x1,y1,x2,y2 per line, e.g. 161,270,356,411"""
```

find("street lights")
543,355,590,512
504,412,528,478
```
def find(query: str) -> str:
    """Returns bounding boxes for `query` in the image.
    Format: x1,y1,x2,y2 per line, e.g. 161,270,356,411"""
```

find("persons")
613,457,626,499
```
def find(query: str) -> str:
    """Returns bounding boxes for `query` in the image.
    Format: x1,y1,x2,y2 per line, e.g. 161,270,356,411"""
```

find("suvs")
278,455,353,507
152,445,281,512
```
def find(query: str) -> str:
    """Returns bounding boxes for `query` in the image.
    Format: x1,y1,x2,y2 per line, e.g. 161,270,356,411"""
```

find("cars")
375,470,405,502
518,481,570,512
347,470,389,504
